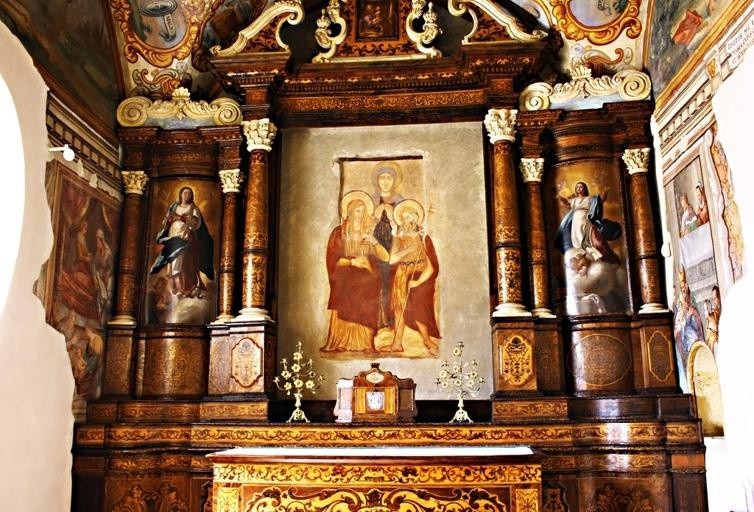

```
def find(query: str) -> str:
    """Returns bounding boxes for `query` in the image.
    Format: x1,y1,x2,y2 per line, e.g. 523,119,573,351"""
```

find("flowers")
272,340,326,395
432,339,487,398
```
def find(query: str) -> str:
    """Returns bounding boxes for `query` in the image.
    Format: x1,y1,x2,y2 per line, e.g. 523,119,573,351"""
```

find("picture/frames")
33,158,123,400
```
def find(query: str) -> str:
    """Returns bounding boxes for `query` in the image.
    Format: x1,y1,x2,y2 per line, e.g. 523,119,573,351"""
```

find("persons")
380,200,441,356
671,261,720,374
321,189,389,357
69,223,100,291
93,227,115,323
671,181,710,236
54,308,104,400
366,161,405,332
557,181,624,266
149,187,215,301
